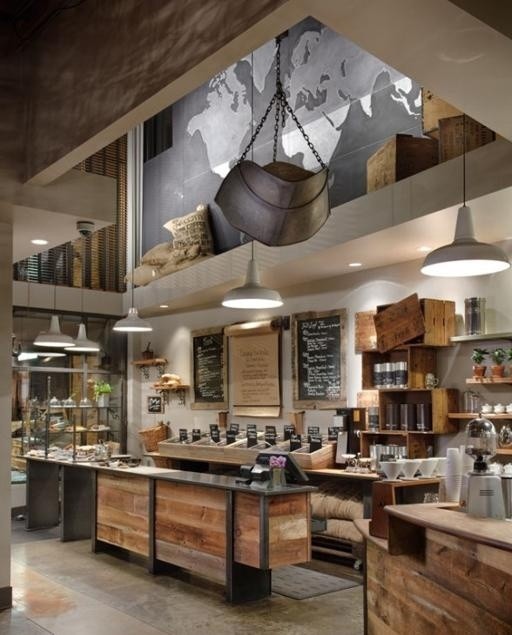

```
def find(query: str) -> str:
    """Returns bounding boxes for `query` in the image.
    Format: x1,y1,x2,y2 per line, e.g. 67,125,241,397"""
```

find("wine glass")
341,454,372,474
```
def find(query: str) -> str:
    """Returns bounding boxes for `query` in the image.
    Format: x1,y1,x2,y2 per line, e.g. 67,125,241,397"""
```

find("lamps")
421,110,509,277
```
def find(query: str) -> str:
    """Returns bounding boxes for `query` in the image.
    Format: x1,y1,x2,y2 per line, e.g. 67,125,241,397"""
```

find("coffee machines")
459,412,507,521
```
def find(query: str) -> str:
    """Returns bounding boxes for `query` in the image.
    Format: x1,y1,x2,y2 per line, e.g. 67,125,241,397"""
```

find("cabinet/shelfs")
143,451,378,573
354,297,512,473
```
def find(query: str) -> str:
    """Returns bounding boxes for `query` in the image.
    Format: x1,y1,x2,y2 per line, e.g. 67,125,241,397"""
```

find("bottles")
373,361,408,389
461,297,486,334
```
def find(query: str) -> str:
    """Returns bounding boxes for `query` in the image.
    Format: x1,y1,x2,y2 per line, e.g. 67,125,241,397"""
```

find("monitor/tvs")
259,451,309,483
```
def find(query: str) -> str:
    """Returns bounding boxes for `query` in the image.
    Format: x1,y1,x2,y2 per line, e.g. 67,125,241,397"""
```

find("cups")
367,406,379,432
385,403,432,431
379,457,449,482
444,446,474,504
369,444,407,472
424,373,439,389
458,391,512,414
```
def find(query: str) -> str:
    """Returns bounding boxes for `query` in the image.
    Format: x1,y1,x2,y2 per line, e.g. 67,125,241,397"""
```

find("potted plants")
92,377,111,407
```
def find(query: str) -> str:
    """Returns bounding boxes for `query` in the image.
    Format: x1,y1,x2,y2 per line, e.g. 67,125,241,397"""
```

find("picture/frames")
290,307,349,409
188,324,230,411
146,396,164,414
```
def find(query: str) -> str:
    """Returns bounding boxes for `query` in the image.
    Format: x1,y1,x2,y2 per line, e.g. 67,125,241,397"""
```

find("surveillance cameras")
77,223,94,239
